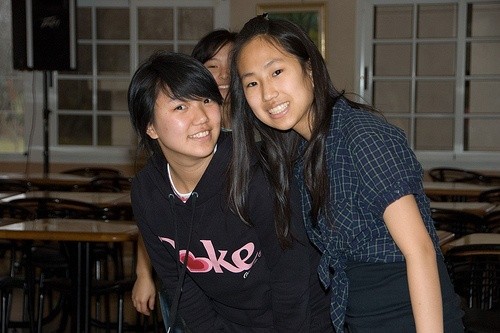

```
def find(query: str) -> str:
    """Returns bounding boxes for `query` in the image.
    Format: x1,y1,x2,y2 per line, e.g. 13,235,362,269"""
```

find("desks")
0,172,115,184
0,191,131,210
0,217,138,333
424,182,500,259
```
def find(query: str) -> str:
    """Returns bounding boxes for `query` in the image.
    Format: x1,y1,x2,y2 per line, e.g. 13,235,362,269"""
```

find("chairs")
478,189,500,201
429,167,500,183
0,167,160,333
430,208,500,232
444,242,500,333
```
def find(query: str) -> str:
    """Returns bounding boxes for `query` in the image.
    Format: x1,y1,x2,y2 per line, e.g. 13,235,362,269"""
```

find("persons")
188,28,239,131
230,15,467,333
127,53,332,333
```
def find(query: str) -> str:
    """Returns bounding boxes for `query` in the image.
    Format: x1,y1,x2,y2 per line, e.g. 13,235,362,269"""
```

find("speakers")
12,0,77,72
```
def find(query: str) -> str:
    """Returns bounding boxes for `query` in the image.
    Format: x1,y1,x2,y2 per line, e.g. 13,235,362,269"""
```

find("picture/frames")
256,0,328,60
